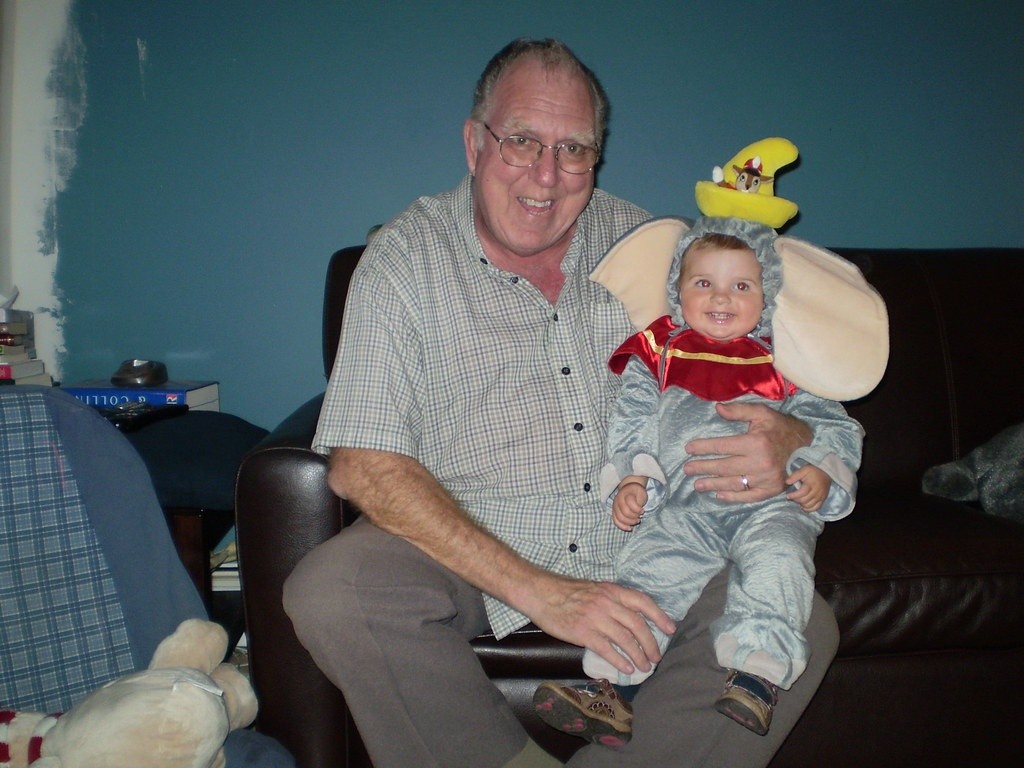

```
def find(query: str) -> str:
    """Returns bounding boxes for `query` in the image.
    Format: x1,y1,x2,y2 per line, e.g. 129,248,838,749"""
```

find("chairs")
0,380,298,766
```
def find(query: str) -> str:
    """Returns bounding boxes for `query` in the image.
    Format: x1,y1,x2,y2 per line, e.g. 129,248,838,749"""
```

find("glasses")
484,123,600,175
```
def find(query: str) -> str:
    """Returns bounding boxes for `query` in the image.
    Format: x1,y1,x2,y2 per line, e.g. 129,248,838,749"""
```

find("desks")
163,508,232,616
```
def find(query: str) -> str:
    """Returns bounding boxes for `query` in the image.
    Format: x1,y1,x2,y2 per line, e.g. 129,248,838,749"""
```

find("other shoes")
711,668,777,736
532,677,635,750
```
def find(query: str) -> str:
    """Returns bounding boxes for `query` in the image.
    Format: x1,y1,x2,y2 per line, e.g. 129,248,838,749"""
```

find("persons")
531,216,866,748
282,36,838,768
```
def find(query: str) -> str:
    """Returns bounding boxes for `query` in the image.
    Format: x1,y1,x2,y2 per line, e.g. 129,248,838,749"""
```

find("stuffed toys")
0,619,259,768
922,422,1024,523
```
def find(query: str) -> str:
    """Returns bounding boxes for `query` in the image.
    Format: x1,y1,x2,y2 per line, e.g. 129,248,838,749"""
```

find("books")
60,378,220,412
212,560,241,592
0,308,52,387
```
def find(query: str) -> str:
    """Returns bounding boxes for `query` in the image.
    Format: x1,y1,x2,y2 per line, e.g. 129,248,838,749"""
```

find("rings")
741,475,750,491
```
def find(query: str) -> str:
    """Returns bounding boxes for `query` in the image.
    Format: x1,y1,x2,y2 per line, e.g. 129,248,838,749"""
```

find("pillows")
126,410,275,513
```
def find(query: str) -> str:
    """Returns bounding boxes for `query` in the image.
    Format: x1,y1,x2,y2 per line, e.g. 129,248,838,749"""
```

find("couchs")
234,246,1024,768
